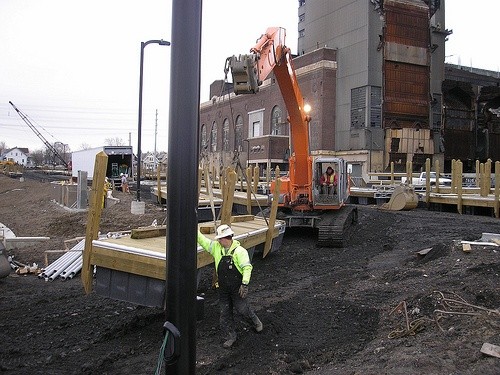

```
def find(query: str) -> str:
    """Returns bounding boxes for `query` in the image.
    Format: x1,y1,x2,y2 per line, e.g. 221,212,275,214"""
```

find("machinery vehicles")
223,26,359,248
9,100,72,176
0,159,17,169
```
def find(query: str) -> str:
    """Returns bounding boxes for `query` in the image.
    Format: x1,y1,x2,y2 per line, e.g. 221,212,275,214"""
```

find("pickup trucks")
402,171,452,192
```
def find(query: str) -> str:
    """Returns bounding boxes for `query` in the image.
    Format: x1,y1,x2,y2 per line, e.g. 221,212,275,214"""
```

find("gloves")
239,285,249,298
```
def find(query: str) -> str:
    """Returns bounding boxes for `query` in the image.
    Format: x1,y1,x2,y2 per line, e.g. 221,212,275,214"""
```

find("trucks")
66,146,134,191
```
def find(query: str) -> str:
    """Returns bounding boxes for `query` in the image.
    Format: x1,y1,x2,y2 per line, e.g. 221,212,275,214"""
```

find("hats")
120,172,124,176
214,224,234,240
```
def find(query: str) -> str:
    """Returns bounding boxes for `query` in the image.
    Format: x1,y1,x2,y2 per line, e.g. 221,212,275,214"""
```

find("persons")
197,224,262,348
319,166,338,194
103,177,109,208
120,173,130,195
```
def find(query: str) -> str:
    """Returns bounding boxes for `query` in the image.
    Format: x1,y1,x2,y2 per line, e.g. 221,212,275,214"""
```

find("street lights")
136,39,171,203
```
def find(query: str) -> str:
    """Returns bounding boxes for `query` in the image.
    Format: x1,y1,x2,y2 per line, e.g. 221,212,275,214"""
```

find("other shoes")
255,321,262,332
223,338,237,348
